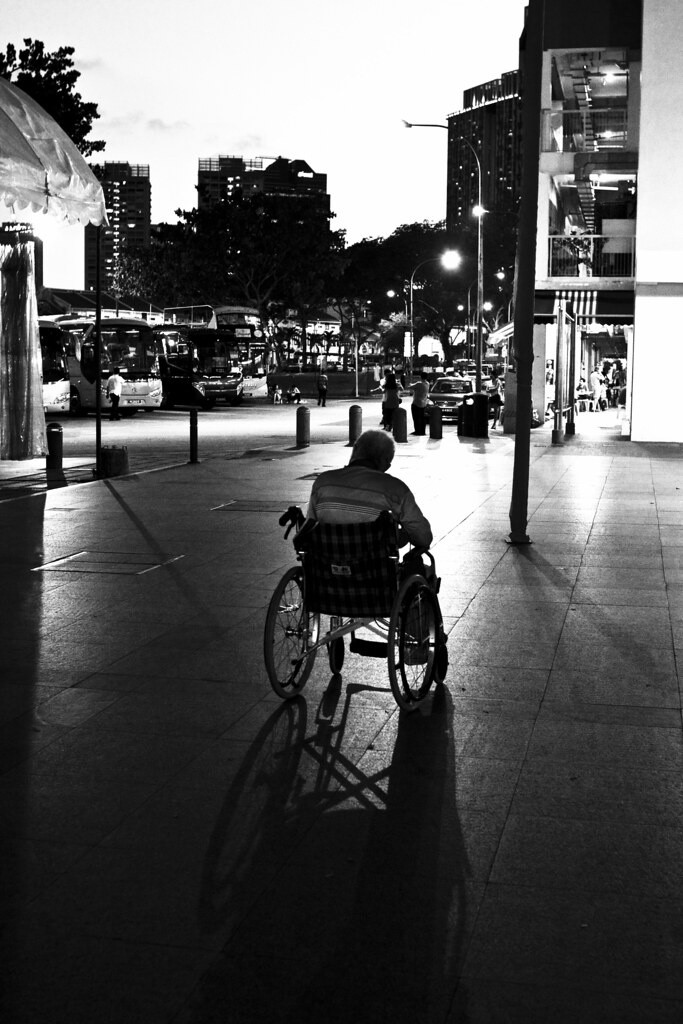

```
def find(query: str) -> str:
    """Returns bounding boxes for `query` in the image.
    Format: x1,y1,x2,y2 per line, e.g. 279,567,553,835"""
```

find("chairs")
441,383,470,393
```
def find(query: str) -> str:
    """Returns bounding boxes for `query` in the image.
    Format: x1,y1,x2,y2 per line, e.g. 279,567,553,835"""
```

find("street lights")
395,119,484,391
410,254,458,383
97,217,137,447
387,291,407,372
472,206,519,319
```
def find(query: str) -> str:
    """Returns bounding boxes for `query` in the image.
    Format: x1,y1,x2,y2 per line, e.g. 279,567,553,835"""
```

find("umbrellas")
0,80,109,227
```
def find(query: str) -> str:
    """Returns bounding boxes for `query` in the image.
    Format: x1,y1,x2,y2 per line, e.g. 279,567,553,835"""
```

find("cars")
429,374,475,419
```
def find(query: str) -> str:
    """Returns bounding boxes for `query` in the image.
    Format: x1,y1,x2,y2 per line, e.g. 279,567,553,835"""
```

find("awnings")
488,320,514,342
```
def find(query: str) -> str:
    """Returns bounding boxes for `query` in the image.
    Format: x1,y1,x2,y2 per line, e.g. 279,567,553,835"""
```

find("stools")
602,398,609,409
617,404,626,418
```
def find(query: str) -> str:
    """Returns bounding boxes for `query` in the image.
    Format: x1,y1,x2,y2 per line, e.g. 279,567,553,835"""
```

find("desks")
578,391,594,412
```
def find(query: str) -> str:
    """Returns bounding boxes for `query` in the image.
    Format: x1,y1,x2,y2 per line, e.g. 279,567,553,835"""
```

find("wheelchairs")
262,504,441,708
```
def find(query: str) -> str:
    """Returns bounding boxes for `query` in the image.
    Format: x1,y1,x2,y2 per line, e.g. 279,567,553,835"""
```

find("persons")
317,369,328,406
274,384,301,404
459,370,467,378
576,361,627,411
306,431,434,663
371,369,404,432
486,369,505,429
106,368,125,421
408,372,429,435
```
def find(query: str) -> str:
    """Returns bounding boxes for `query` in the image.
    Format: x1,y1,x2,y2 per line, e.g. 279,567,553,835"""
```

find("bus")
43,314,163,414
152,326,244,410
37,319,81,412
165,304,268,399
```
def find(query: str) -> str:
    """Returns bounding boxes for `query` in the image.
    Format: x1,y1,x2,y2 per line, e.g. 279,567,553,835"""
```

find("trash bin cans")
463,393,489,439
46,423,63,468
